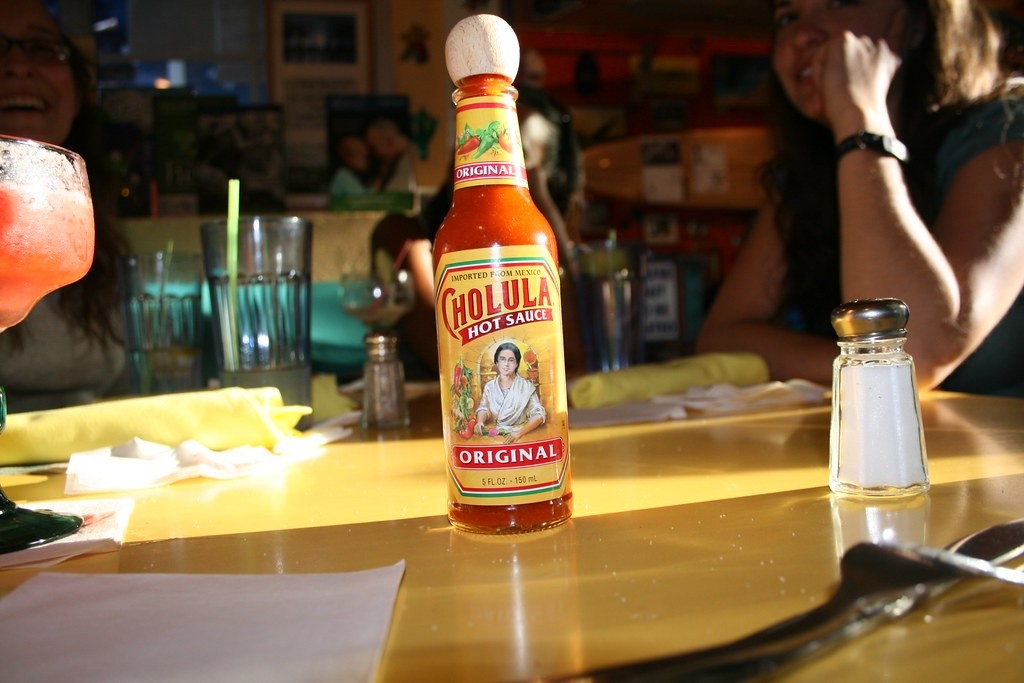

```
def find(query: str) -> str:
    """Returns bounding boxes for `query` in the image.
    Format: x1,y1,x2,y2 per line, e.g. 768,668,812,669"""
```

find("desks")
0,378,1024,683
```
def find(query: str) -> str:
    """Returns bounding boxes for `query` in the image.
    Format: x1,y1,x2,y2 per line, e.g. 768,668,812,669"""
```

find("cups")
571,241,634,375
339,268,413,330
198,215,313,431
118,249,210,395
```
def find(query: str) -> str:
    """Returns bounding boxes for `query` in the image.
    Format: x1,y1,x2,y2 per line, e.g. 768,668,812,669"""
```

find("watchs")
835,132,909,162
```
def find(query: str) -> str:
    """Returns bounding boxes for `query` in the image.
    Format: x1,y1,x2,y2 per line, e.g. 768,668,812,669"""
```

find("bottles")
360,333,413,430
828,297,932,501
431,74,576,535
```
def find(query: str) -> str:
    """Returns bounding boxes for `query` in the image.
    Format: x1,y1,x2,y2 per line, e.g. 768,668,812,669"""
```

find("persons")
192,111,282,214
0,0,130,415
368,49,587,383
695,0,1024,397
329,118,418,196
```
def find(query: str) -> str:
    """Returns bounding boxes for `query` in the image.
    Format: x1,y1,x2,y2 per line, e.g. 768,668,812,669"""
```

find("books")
0,497,134,568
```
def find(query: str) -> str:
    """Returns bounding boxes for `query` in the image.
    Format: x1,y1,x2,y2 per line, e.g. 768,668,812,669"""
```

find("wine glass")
0,134,94,553
